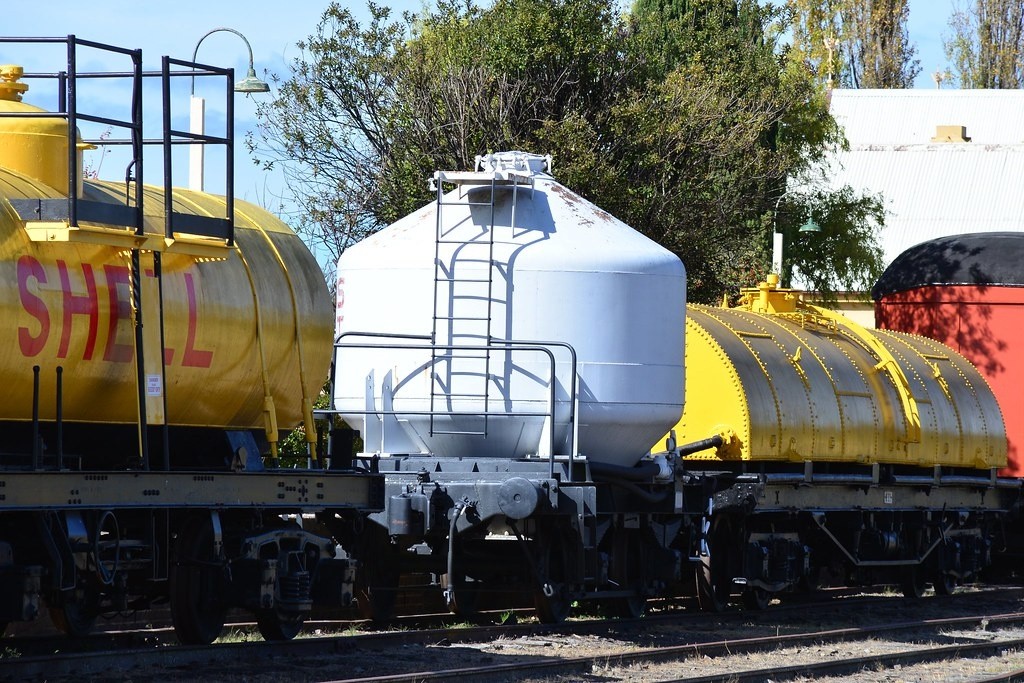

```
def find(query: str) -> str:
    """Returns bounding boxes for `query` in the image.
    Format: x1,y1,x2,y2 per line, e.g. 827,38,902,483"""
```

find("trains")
0,35,1024,655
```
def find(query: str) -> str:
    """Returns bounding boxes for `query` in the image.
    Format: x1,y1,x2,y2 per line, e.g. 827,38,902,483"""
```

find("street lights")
771,193,820,289
187,27,270,192
